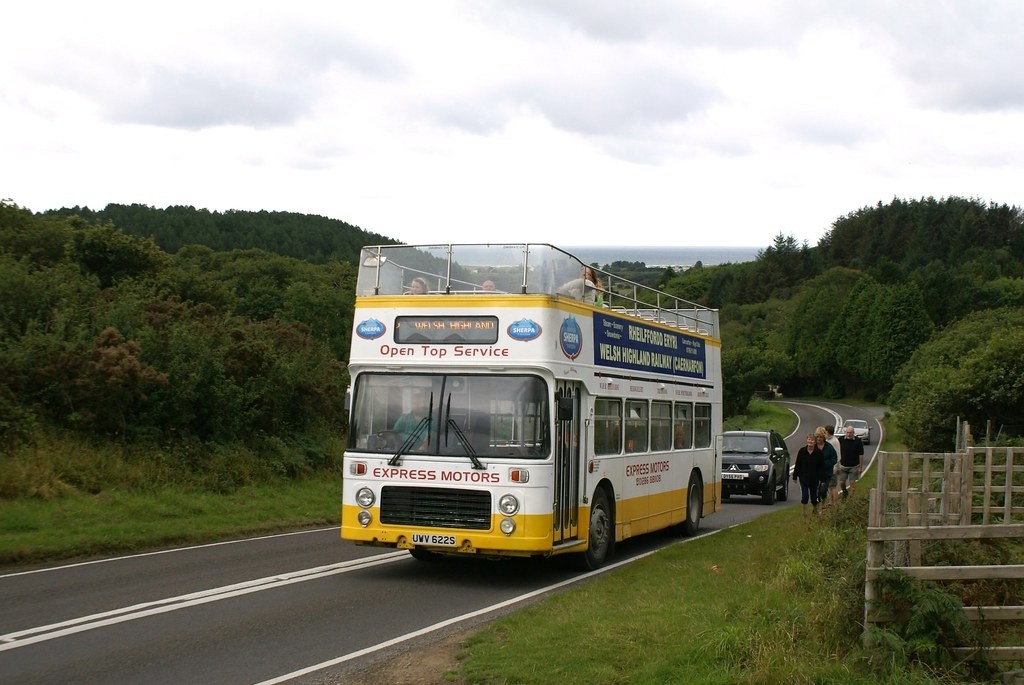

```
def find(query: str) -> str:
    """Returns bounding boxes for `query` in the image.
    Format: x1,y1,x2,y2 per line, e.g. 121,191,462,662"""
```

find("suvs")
721,429,791,505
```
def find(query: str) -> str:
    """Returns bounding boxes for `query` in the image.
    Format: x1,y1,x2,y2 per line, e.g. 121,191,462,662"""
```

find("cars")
842,418,873,444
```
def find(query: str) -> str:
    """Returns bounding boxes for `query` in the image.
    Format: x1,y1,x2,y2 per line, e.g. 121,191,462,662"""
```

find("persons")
482,280,495,291
411,278,428,295
653,432,668,450
557,267,598,304
813,426,837,500
838,426,864,490
594,278,604,308
793,434,826,506
674,424,685,449
392,393,434,453
825,424,842,482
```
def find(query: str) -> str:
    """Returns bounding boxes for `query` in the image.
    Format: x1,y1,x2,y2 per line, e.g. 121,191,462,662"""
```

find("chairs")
365,294,712,459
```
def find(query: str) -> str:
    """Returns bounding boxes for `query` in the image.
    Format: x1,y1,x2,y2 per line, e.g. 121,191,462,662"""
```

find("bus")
338,241,726,580
